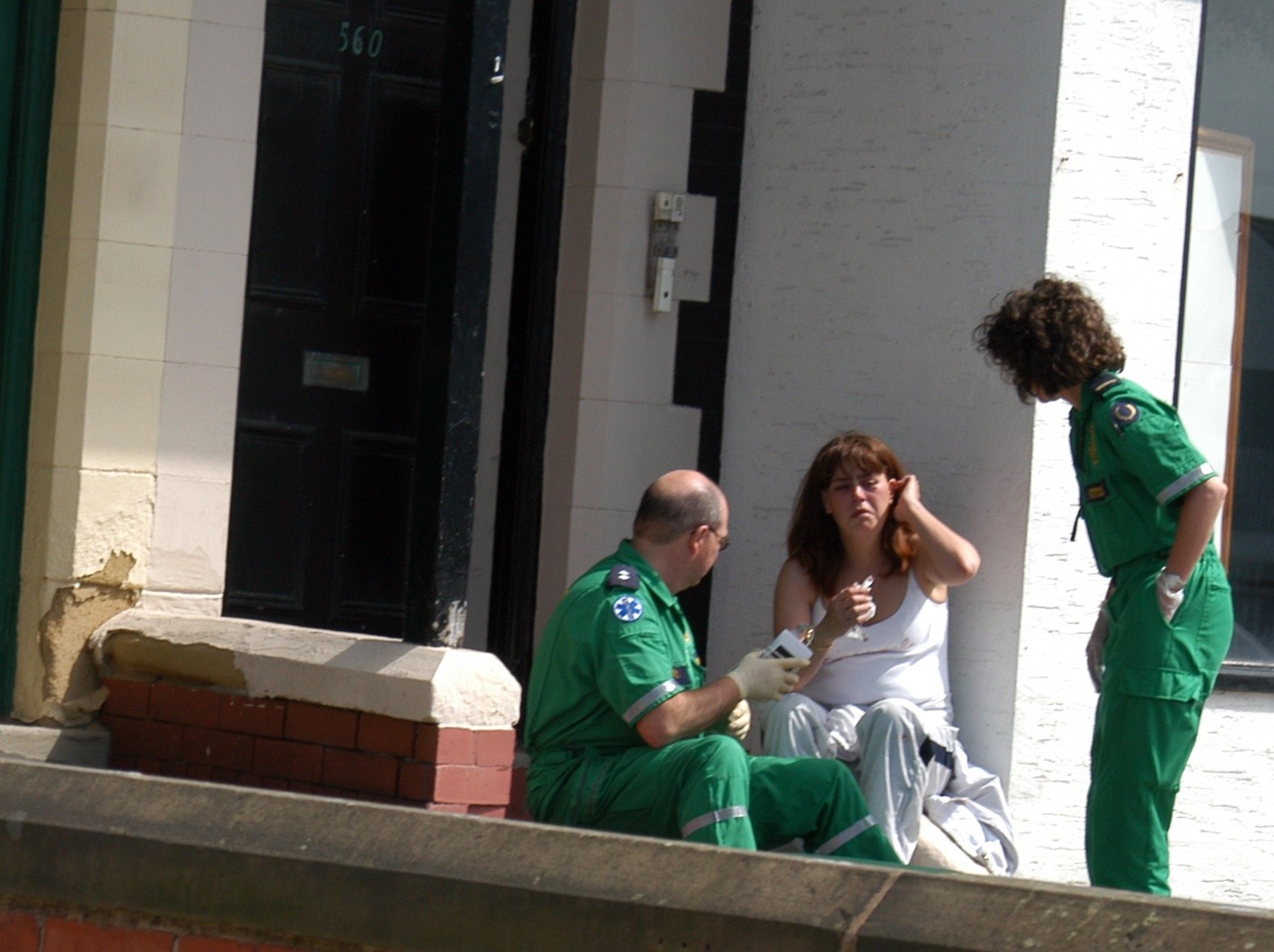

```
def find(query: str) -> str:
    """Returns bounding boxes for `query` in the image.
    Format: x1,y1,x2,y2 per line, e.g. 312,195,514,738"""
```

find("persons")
971,273,1236,897
523,467,905,866
760,430,982,866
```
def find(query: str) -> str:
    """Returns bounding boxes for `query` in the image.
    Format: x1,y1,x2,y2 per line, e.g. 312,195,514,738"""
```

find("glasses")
691,526,729,551
